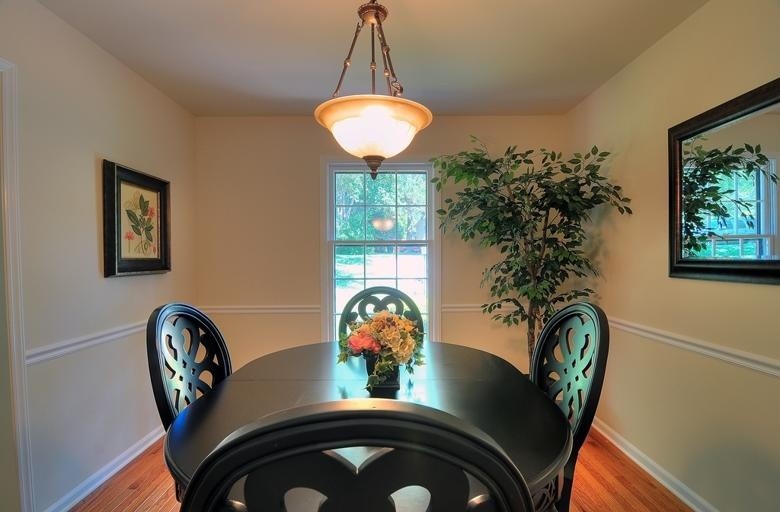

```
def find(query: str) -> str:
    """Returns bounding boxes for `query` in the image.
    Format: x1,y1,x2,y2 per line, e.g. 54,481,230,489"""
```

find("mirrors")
668,77,780,284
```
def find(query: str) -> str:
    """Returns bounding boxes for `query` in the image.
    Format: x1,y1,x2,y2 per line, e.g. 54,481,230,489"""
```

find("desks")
164,341,574,511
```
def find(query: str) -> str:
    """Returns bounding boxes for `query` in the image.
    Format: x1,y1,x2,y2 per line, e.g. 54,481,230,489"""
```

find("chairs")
530,301,609,511
146,301,233,434
338,285,424,344
178,398,537,511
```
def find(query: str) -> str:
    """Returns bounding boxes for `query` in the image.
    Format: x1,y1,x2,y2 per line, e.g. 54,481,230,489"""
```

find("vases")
364,356,401,392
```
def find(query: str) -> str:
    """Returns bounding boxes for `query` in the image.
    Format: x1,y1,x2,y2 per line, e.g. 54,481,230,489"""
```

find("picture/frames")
100,158,172,279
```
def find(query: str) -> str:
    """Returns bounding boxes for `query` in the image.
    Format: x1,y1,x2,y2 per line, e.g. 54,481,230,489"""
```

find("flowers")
337,309,427,392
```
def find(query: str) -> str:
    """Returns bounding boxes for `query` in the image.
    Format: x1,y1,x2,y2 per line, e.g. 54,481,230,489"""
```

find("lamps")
313,1,434,180
368,217,394,230
